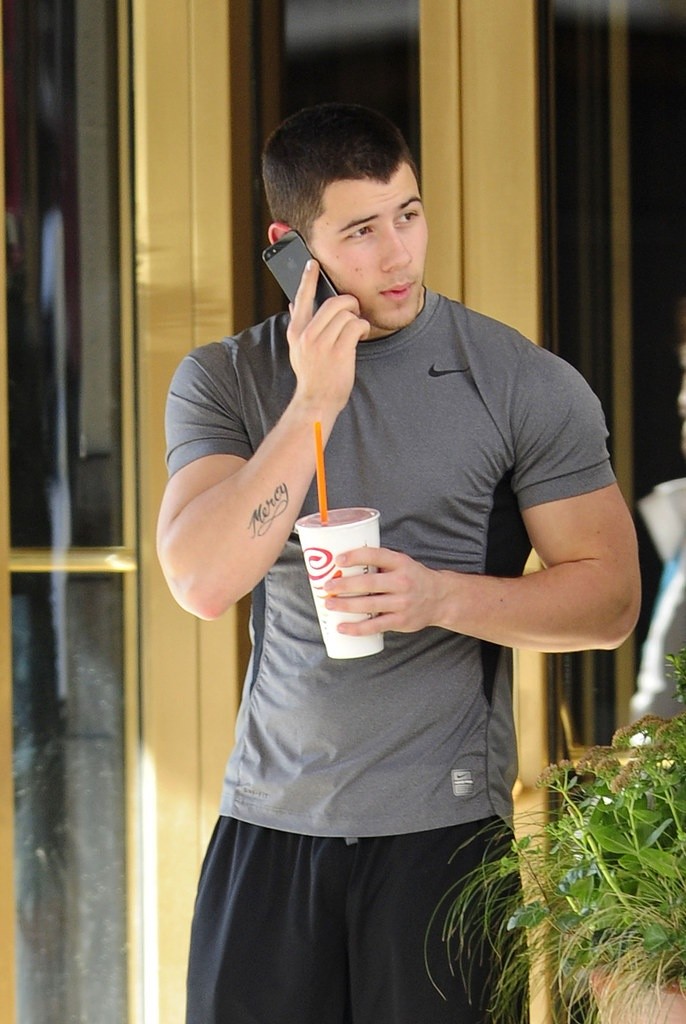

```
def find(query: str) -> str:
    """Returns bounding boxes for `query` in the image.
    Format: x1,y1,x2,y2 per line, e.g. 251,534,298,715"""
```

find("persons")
554,340,686,981
155,104,643,1023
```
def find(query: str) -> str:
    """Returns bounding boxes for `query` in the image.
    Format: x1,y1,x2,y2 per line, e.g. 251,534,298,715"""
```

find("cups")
295,505,384,658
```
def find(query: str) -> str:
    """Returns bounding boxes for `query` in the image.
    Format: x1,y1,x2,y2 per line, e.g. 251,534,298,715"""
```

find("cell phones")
262,229,339,316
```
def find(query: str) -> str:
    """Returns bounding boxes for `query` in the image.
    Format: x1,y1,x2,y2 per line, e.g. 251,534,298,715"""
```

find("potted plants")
425,644,685,1024
